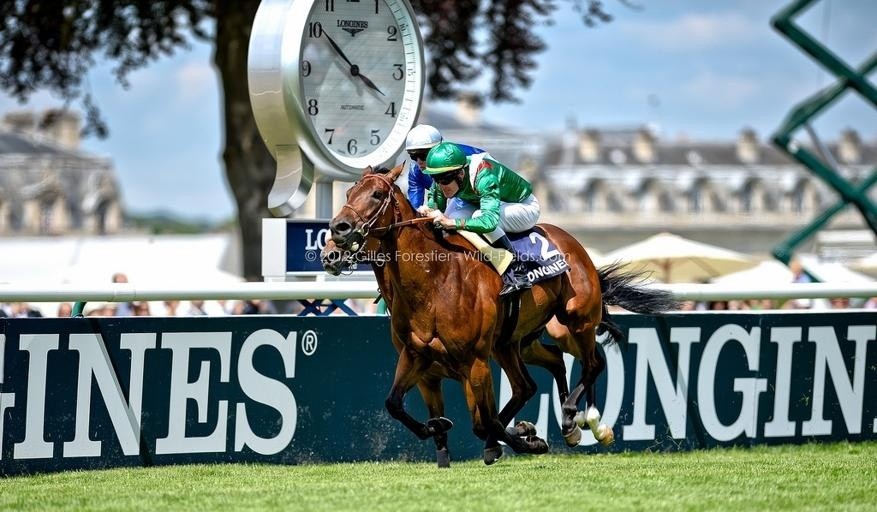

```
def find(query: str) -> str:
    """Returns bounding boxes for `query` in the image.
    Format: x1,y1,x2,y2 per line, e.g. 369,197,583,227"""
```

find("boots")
492,235,533,297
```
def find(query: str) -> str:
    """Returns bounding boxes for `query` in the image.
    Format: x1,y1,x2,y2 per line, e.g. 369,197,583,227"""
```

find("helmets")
405,124,442,151
422,142,468,175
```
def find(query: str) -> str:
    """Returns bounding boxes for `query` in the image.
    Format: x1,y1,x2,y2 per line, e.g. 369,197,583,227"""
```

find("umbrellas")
585,233,877,285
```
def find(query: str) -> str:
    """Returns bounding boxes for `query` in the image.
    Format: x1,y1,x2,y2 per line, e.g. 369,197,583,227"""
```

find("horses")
319,238,631,468
328,160,685,456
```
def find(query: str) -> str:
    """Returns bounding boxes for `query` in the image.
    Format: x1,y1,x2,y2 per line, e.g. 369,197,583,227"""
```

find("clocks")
247,0,425,218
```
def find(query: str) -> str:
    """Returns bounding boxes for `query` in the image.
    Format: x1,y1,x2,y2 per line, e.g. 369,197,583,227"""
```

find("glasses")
434,174,457,186
410,153,427,161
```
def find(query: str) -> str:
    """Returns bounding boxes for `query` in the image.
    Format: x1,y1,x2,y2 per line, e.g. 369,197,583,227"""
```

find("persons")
403,122,489,212
416,141,541,298
0,273,876,319
